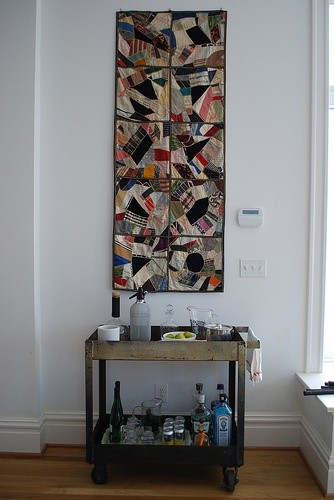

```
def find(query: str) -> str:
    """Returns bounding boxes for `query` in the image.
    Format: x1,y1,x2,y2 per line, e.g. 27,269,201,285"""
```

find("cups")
98,325,120,341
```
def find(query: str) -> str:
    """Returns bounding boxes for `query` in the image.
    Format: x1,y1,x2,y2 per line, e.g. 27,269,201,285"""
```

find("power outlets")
154,382,168,403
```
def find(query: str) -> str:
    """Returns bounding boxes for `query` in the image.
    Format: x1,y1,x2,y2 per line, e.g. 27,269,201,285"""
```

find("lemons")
166,331,192,339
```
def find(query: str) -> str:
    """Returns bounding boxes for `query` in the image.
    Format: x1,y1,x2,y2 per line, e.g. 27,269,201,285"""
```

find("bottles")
129,287,151,341
109,381,123,443
211,384,228,437
192,394,213,440
105,291,126,334
161,305,178,340
194,383,206,408
214,394,232,446
194,419,209,447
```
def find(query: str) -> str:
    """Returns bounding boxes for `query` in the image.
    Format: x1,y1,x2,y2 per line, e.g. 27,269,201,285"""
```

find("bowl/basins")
204,324,236,342
163,331,196,341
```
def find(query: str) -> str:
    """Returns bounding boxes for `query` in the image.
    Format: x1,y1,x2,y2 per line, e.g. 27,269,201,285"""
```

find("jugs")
133,398,163,440
187,306,219,341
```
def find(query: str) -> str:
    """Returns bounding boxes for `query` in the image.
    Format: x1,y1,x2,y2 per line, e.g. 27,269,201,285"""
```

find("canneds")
162,416,184,445
